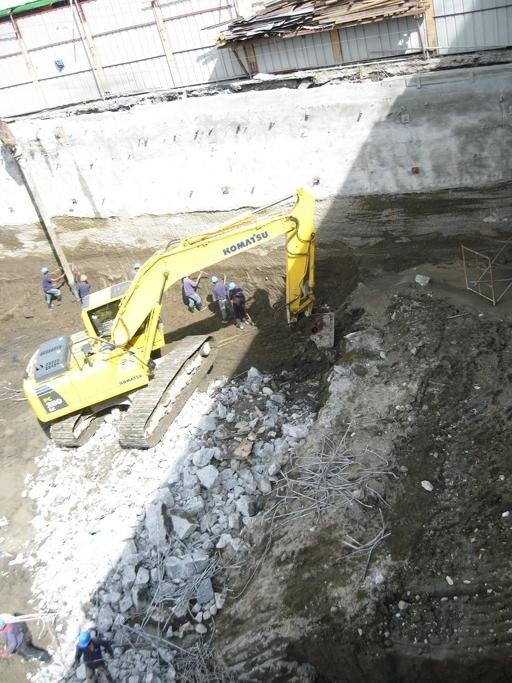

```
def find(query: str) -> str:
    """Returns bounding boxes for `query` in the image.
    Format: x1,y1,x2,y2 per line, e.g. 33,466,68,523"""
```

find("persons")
72,631,116,683
75,271,91,298
131,262,142,278
183,271,207,312
229,283,247,329
211,273,228,324
0,618,54,663
41,267,66,307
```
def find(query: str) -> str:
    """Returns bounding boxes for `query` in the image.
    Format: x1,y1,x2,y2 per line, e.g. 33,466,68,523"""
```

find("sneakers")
191,305,208,312
48,301,63,309
221,320,247,328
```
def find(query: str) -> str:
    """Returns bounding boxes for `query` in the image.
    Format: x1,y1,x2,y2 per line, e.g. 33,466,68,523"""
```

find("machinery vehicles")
23,188,336,450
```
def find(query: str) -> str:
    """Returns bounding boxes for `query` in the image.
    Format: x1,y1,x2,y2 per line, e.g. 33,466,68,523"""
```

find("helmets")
80,275,88,282
211,276,218,283
41,267,49,274
0,618,5,628
133,263,142,269
79,631,92,649
228,283,236,290
